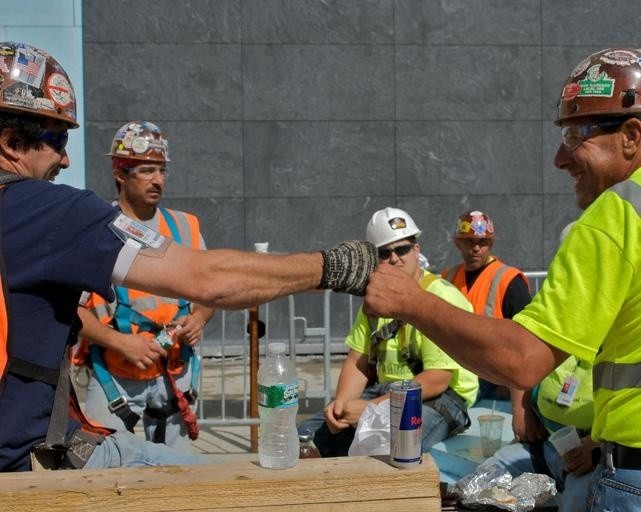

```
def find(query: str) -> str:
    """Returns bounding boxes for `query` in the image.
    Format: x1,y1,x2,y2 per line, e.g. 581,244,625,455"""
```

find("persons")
75,120,216,452
456,222,600,512
440,210,533,400
0,40,378,471
297,206,480,454
362,46,640,512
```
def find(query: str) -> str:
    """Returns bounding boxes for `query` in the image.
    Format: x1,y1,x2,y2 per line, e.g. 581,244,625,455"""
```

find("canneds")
388,379,423,468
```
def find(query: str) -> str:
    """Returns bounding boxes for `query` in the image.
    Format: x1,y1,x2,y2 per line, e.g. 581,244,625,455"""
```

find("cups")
477,415,506,456
548,423,584,459
254,242,269,253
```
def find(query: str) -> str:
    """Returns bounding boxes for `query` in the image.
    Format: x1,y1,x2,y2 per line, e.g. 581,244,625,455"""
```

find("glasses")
560,120,625,151
375,242,417,260
122,164,170,180
36,131,70,154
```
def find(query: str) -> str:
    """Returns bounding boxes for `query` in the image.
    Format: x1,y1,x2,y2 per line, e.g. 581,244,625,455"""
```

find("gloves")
321,241,379,297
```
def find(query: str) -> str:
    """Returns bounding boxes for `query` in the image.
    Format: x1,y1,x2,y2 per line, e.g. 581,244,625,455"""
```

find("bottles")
152,324,183,351
252,342,302,474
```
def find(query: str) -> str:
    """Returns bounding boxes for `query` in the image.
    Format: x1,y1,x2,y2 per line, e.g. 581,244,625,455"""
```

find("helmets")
448,211,502,244
0,40,80,130
552,47,641,126
101,120,171,163
364,205,422,248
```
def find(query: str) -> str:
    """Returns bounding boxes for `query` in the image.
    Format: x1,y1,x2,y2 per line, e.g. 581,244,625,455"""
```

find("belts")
598,442,640,473
